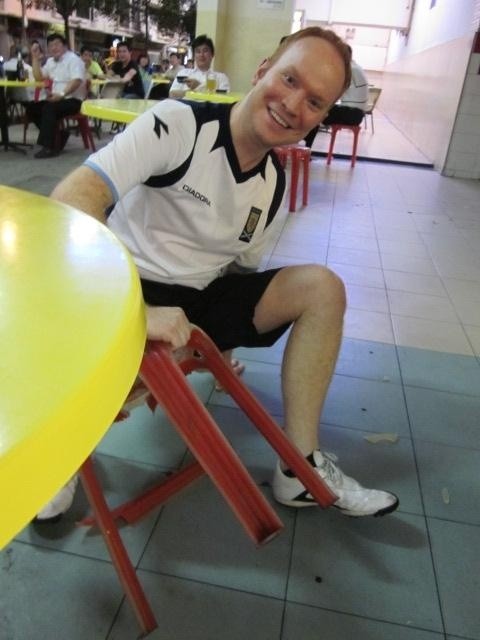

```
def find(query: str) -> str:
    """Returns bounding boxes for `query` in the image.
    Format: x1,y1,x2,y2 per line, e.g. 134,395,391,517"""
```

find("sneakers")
271,449,400,520
32,475,80,524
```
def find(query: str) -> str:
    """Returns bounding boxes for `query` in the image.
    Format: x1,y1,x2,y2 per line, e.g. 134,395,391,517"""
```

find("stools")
274,144,312,212
326,125,362,167
80,324,340,636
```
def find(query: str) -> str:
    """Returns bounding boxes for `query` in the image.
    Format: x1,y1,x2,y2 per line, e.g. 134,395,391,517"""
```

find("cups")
207,73,217,97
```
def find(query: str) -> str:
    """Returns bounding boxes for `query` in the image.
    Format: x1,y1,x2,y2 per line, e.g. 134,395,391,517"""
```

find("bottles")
17,51,25,81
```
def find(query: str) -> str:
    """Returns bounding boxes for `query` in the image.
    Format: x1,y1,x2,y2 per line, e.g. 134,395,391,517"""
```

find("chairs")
364,88,382,135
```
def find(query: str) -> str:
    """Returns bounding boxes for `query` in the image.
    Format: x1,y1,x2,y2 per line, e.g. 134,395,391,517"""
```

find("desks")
0,79,242,155
0,184,147,551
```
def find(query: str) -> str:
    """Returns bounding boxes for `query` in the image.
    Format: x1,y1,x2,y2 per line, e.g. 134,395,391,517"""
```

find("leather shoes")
34,128,70,158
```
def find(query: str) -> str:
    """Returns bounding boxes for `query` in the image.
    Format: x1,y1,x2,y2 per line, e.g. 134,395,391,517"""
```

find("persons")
0,32,230,159
303,44,370,161
33,28,399,526
